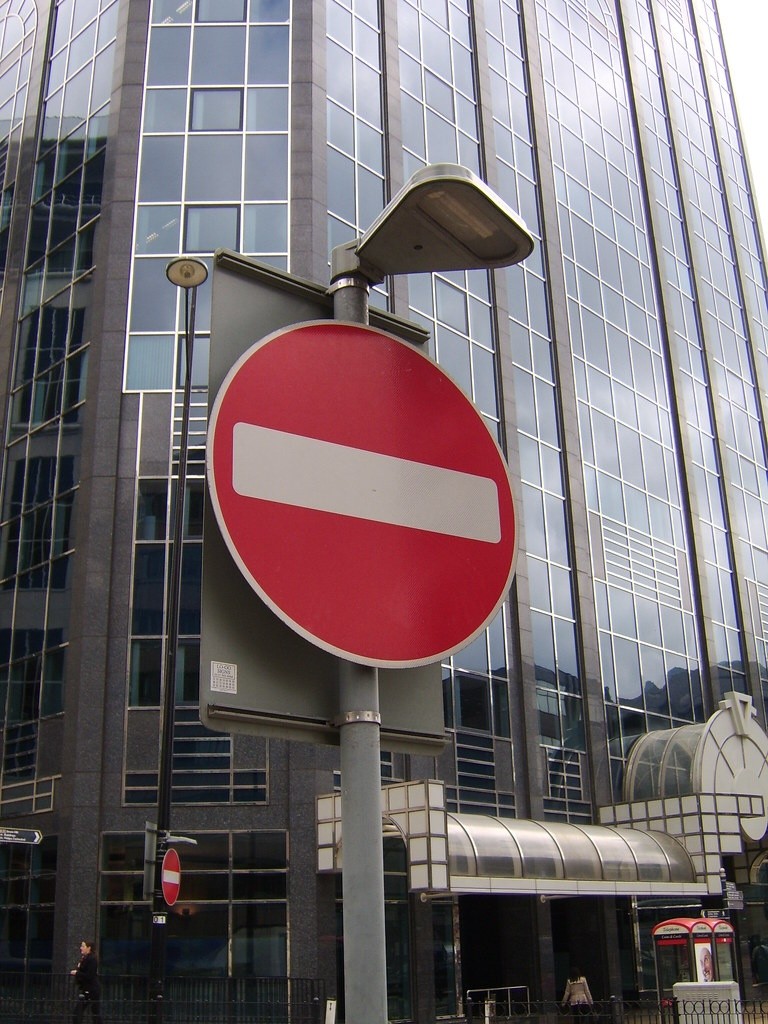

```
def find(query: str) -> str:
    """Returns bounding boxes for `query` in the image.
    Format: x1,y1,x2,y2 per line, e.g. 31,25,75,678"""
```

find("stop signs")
159,849,182,906
204,321,521,671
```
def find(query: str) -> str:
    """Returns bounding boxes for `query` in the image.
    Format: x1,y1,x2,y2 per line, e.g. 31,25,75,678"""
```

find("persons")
562,965,594,1024
699,947,714,982
70,940,101,1024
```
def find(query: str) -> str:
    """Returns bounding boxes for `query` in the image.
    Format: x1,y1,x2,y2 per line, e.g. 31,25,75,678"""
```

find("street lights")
327,162,536,1024
149,257,213,1023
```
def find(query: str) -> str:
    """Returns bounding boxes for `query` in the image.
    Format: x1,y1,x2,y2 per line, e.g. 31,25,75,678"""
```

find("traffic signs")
0,828,44,844
